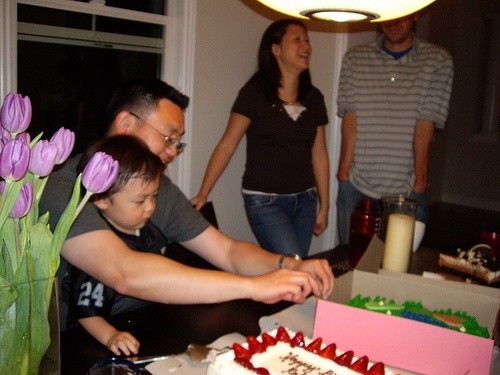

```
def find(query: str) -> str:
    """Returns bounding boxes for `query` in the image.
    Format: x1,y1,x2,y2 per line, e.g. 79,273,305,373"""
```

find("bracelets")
278,252,301,270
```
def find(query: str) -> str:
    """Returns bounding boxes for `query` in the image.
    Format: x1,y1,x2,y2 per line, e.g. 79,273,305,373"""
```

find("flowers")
0,92,119,287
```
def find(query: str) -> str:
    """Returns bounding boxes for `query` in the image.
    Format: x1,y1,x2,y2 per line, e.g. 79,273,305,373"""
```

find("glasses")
128,109,187,153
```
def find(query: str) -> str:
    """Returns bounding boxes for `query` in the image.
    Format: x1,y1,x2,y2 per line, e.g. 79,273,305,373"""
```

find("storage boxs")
312,270,500,375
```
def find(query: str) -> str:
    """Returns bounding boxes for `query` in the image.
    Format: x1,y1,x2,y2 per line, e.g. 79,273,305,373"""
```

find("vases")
0,276,60,375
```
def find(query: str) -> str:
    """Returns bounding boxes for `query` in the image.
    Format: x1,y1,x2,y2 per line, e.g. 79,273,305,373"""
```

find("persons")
190,19,330,260
337,12,454,247
43,78,336,356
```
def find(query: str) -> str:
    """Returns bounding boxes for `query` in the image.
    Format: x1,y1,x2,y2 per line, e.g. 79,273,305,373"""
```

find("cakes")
206,325,403,375
344,294,490,338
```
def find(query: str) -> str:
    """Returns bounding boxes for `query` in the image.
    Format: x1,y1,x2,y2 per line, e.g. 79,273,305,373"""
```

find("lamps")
258,0,436,22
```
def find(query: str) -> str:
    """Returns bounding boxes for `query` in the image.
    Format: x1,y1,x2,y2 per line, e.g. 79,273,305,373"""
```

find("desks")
61,243,500,375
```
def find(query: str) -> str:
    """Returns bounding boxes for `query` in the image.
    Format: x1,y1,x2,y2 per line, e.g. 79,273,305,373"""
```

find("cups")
412,220,426,252
382,198,417,274
